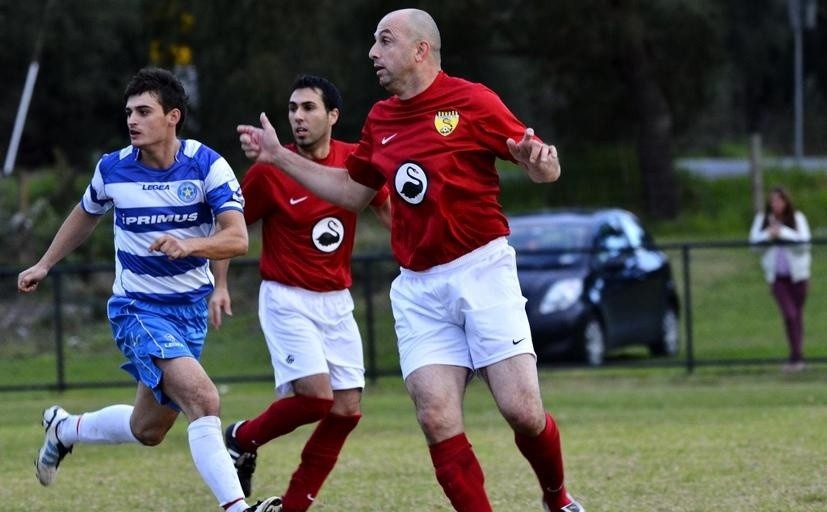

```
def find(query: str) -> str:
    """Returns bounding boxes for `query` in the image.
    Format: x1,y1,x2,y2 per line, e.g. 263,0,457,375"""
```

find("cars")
506,208,681,368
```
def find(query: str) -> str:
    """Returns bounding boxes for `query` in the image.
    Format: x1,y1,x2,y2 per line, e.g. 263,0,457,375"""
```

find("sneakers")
34,405,73,486
223,420,256,497
243,496,283,512
541,491,585,512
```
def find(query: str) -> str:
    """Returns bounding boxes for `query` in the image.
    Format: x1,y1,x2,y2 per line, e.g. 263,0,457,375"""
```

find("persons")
235,7,589,512
206,72,393,512
745,185,814,374
15,64,286,512
